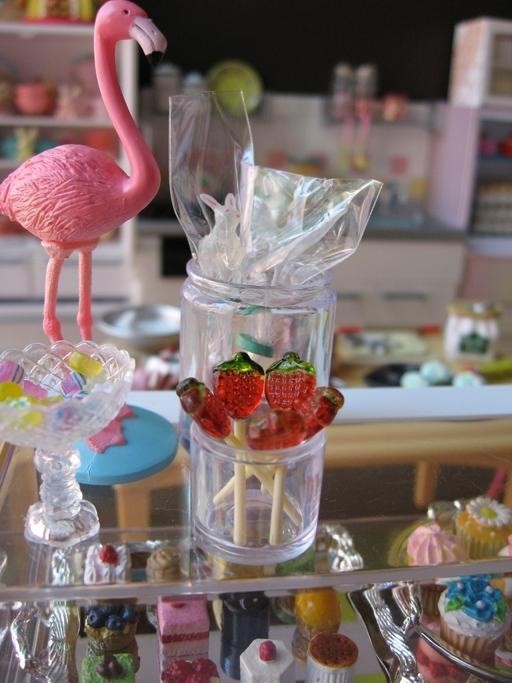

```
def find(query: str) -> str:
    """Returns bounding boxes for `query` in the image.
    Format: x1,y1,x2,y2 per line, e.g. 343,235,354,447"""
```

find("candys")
179,351,344,546
0,351,102,427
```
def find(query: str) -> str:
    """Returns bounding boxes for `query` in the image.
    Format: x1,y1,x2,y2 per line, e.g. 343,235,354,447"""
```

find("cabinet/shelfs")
2,17,132,292
324,244,463,316
434,16,512,316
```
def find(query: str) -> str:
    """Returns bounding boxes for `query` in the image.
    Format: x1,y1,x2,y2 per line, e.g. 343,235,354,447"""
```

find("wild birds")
0,0,169,455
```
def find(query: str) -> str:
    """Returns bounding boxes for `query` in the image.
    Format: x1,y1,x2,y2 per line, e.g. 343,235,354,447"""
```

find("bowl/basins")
13,81,54,116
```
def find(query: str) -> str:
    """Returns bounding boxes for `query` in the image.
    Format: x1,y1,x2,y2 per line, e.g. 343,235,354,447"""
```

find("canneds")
443,301,501,359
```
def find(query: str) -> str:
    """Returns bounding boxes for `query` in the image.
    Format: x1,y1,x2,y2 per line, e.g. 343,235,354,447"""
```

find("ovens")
133,230,199,306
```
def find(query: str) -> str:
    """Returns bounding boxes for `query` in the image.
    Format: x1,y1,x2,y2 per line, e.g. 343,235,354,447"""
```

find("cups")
176,256,334,418
185,422,330,567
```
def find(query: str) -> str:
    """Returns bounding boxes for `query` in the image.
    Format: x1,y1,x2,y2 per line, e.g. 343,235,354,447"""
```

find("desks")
109,328,512,544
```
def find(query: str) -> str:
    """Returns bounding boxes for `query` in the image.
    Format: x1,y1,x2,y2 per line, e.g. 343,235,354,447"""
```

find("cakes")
406,497,512,683
82,543,359,683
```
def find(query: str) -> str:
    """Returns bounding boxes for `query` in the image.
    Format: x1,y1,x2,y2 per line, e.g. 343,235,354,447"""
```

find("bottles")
444,302,496,365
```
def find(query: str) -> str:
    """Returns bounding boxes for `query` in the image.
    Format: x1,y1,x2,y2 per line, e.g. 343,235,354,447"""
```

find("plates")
208,60,263,118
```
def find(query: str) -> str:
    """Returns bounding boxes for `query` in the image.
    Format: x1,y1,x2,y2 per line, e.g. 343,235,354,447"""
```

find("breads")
400,361,484,391
